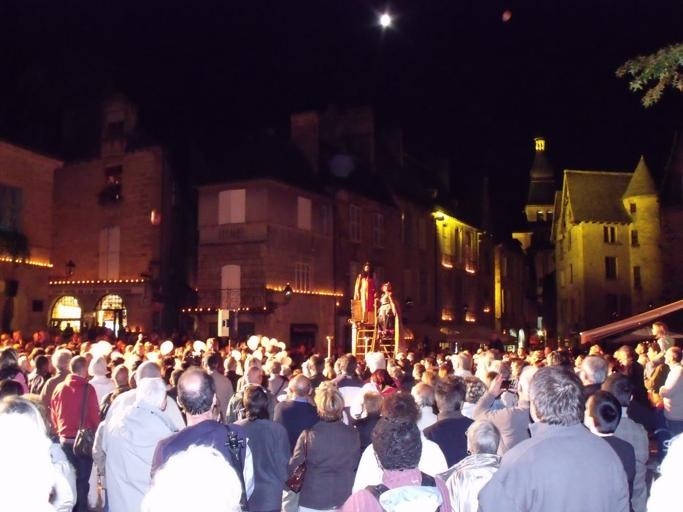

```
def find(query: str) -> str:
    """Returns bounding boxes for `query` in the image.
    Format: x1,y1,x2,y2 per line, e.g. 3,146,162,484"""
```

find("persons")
374,280,397,335
353,261,374,323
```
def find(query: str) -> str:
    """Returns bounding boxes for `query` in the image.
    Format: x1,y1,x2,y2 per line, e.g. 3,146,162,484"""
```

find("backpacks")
365,471,445,512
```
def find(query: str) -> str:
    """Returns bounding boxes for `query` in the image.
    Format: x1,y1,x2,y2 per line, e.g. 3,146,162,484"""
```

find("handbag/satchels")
284,462,308,494
72,426,96,459
